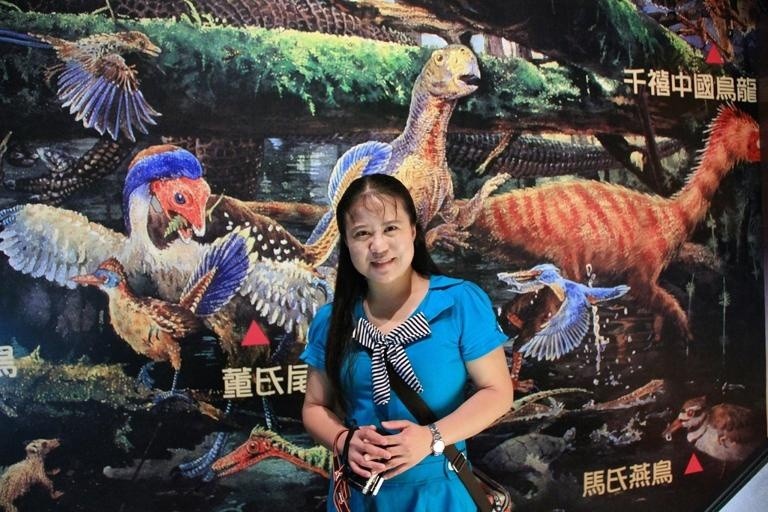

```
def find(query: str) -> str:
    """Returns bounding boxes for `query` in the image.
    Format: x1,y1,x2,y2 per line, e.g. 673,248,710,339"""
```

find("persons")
298,172,515,512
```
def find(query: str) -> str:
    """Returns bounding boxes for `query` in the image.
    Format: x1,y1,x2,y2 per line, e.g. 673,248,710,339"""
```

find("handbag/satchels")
469,465,512,512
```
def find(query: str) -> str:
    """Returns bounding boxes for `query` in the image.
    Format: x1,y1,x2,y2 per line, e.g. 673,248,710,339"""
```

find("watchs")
426,422,445,458
